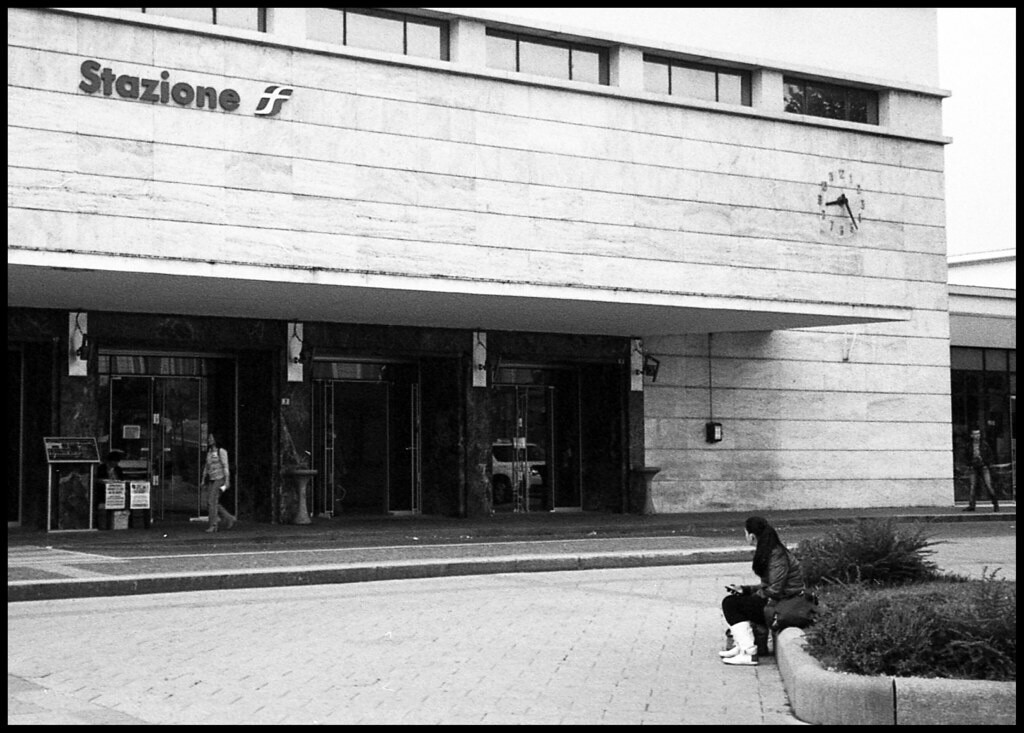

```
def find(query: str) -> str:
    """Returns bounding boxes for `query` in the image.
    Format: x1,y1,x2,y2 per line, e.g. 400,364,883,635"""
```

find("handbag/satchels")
761,586,819,631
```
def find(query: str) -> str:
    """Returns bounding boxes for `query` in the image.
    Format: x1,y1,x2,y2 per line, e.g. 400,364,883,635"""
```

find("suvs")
491,443,546,505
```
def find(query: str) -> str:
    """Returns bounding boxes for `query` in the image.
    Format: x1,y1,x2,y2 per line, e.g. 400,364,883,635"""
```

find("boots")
720,620,758,665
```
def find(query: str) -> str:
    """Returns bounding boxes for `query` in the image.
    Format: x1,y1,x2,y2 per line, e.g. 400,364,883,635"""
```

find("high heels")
205,525,218,533
226,516,237,529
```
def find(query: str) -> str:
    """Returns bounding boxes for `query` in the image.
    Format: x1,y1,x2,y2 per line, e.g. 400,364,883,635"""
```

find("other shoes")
963,503,975,511
994,501,999,511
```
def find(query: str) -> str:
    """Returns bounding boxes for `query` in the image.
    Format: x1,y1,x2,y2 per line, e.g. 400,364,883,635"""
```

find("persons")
718,516,805,665
962,426,1000,512
201,433,236,533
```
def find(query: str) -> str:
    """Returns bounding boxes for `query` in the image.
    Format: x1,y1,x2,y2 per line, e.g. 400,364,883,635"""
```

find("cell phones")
725,586,742,596
